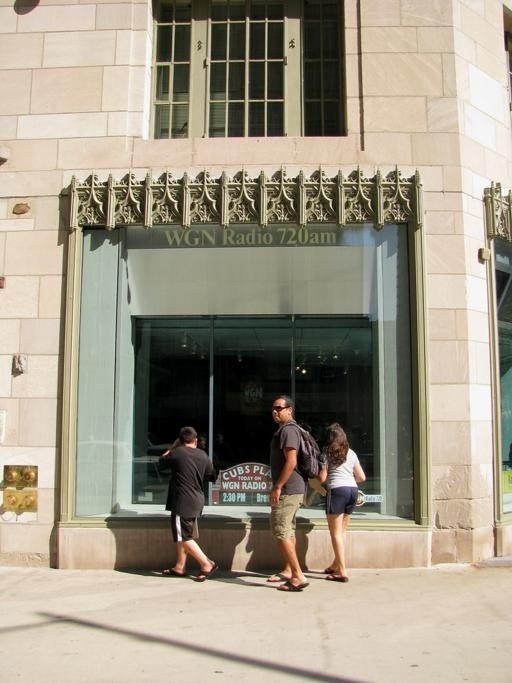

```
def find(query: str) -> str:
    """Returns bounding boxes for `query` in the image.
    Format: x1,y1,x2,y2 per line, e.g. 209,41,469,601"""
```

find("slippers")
162,568,187,577
269,572,291,583
326,574,349,583
195,562,218,582
325,567,336,574
277,580,310,593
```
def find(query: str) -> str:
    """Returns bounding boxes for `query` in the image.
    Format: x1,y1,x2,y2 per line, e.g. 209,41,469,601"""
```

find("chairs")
133,439,172,483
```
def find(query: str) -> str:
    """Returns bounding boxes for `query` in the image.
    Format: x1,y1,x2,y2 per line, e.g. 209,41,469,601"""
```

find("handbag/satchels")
278,422,321,480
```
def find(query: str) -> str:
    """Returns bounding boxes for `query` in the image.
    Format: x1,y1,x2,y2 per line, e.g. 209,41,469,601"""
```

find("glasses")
274,406,290,411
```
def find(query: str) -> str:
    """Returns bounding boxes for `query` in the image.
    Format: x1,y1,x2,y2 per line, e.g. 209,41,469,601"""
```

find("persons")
158,426,219,582
269,393,310,591
318,422,366,583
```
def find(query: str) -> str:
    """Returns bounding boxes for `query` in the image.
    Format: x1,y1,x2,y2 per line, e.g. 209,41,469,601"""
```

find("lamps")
237,349,242,362
181,331,208,360
